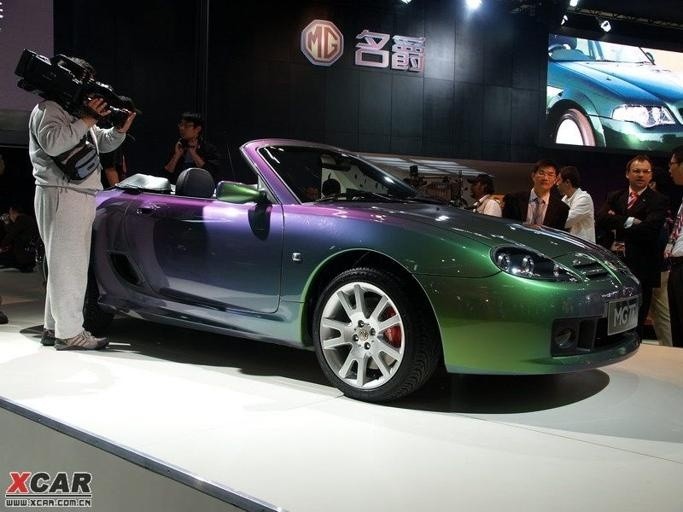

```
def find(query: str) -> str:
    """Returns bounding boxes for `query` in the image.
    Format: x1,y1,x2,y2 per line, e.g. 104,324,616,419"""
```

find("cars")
545,35,683,155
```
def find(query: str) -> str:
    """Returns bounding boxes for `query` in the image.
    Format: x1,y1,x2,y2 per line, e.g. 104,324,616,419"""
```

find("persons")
161,111,214,185
0,207,37,273
306,184,319,201
96,96,142,190
29,57,137,351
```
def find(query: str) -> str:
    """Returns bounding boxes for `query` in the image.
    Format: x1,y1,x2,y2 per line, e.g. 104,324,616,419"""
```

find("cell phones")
179,140,188,148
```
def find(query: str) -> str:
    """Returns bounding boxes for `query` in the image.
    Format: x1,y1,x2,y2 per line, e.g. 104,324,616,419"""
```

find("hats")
467,174,493,186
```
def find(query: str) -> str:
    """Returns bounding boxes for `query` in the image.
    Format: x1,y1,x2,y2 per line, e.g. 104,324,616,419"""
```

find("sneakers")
43,330,110,350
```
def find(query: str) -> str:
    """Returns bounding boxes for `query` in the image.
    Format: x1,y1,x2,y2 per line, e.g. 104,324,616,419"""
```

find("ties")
530,197,544,225
626,192,638,210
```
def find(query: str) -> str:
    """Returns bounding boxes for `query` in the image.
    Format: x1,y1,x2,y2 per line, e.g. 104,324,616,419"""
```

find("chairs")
174,165,216,199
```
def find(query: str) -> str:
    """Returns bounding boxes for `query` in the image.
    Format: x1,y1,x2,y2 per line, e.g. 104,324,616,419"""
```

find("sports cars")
78,134,649,409
